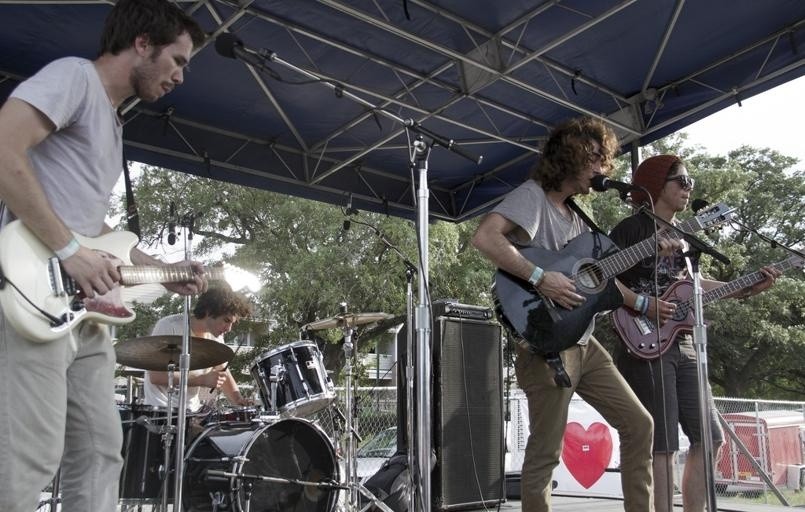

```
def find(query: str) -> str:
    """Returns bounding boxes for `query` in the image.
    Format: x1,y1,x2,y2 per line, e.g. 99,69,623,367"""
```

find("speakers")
396,316,505,512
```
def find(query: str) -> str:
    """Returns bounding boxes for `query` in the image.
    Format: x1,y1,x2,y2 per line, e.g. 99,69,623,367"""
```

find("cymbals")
302,313,393,330
114,335,233,371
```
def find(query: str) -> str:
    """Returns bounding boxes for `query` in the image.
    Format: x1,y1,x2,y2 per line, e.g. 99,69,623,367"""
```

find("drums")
250,338,336,418
183,416,340,511
117,406,190,503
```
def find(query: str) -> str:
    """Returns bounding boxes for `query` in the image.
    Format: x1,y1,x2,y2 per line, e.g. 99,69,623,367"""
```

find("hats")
631,155,678,204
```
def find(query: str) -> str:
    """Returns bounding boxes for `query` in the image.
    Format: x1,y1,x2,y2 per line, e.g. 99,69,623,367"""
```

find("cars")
114,387,138,402
336,424,397,507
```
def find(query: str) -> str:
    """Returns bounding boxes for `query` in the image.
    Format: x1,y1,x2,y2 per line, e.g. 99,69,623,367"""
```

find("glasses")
667,175,695,189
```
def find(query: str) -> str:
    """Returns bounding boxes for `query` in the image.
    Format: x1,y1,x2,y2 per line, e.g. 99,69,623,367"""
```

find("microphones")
692,199,708,211
169,201,175,244
215,33,280,80
592,174,644,192
343,193,352,229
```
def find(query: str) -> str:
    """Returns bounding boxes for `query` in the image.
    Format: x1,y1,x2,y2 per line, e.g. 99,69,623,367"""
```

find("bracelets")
743,288,751,300
634,293,649,316
56,238,83,260
527,266,544,286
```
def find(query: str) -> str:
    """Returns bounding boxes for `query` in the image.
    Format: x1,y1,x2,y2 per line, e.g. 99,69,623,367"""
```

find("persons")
0,1,208,510
607,155,782,512
471,116,684,512
143,289,252,415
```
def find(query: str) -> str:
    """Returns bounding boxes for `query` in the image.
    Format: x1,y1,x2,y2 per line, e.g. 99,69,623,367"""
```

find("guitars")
0,219,228,344
489,204,733,355
613,250,805,360
